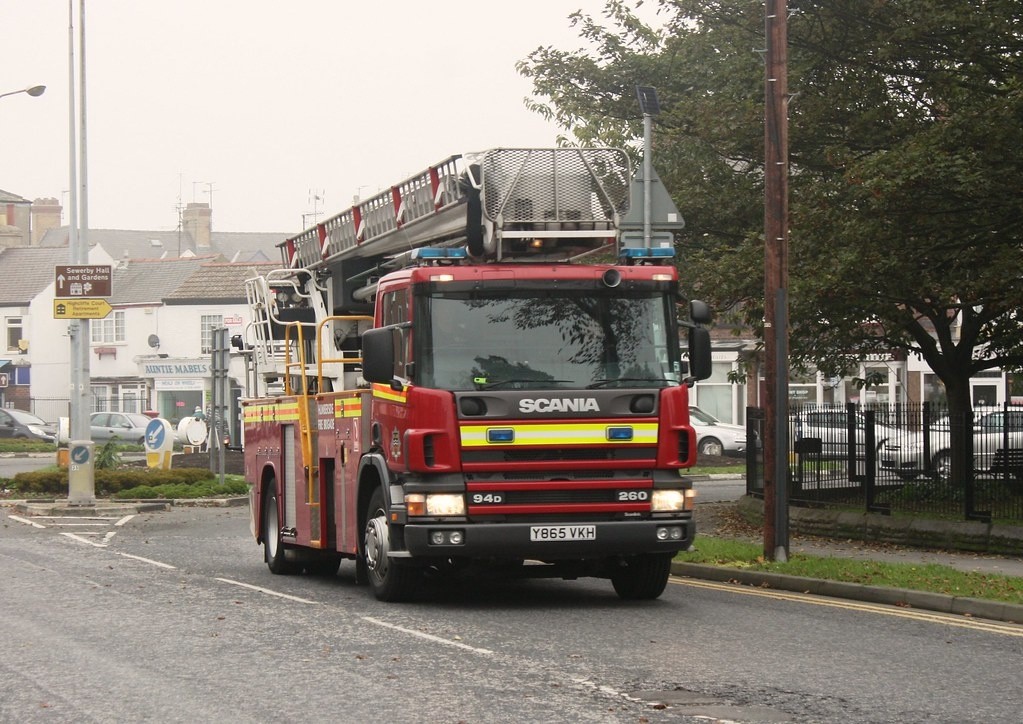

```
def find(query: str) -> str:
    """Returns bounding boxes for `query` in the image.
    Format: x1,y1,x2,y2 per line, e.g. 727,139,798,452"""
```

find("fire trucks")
231,147,712,602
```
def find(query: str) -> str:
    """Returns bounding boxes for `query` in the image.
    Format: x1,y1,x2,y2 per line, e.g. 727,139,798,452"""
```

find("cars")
789,406,1023,480
689,405,746,458
91,412,179,445
1,408,57,443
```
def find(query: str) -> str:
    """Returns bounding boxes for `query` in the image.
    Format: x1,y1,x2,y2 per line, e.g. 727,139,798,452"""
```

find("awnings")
0,360,11,369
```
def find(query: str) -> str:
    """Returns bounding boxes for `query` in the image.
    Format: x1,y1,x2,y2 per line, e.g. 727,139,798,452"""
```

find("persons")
192,405,205,419
